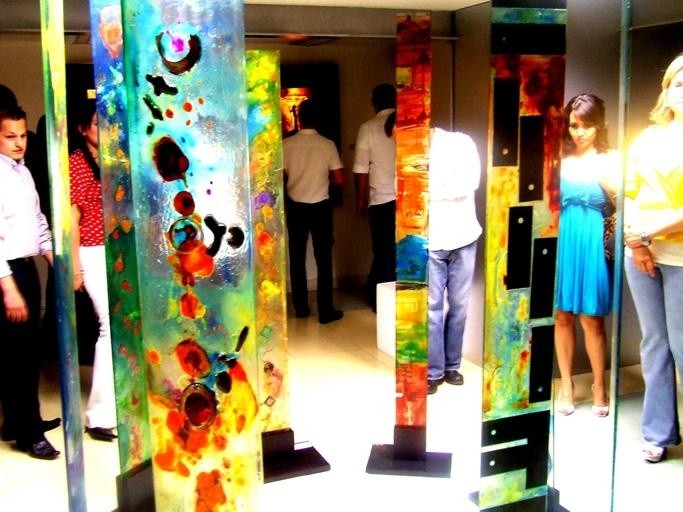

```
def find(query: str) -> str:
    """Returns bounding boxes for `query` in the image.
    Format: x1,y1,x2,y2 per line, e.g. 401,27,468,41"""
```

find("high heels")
642,443,667,463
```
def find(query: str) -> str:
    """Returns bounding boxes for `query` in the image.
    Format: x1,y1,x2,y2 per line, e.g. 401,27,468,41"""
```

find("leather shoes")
318,311,343,324
427,378,444,394
0,418,60,441
85,426,117,440
15,437,59,459
445,369,463,385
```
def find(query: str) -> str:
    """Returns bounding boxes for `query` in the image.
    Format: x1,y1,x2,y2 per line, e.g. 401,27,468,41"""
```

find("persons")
623,52,683,463
283,97,346,324
1,85,116,459
554,93,617,418
428,127,482,397
352,83,396,310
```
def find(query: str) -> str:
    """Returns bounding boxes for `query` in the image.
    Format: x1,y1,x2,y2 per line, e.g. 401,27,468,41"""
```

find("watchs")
640,230,651,246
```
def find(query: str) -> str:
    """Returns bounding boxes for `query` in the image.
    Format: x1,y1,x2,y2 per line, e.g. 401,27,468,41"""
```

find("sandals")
557,390,574,416
591,384,609,418
